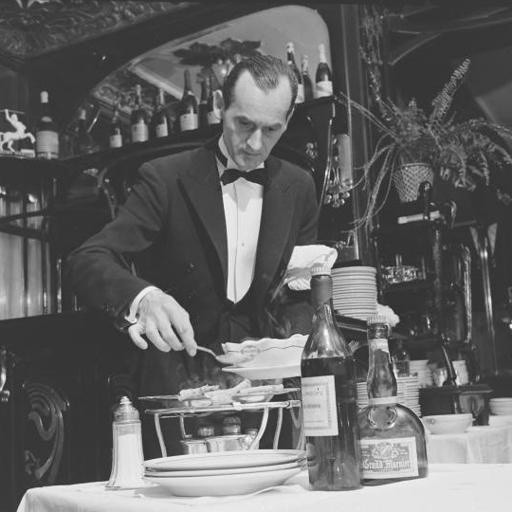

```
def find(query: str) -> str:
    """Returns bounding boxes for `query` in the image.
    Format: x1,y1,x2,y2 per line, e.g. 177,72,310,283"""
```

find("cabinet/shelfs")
369,182,465,348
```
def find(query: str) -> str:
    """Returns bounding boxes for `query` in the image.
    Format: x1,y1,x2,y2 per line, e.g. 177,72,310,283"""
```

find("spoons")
180,342,254,364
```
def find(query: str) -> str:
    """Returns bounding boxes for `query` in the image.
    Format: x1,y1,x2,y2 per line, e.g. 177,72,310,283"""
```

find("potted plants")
331,57,512,253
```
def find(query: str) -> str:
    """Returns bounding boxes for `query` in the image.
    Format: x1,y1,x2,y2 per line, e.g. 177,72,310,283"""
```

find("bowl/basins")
420,412,474,435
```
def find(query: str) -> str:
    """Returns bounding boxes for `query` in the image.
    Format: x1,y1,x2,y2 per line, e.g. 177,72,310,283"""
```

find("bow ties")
221,168,266,186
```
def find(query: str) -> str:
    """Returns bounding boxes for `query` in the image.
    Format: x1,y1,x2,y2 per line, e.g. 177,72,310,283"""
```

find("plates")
143,449,308,496
488,396,511,416
408,359,429,388
355,376,422,420
222,361,301,380
330,265,378,319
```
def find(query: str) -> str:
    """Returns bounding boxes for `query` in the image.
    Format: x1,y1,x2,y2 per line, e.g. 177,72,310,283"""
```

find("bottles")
36,90,59,158
107,395,147,488
154,88,172,138
74,109,94,158
299,273,360,490
108,110,123,149
178,70,199,133
129,84,150,144
357,322,429,486
284,42,332,103
201,77,221,132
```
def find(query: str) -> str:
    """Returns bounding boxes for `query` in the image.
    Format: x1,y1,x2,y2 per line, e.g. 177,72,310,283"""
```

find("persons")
60,55,320,462
